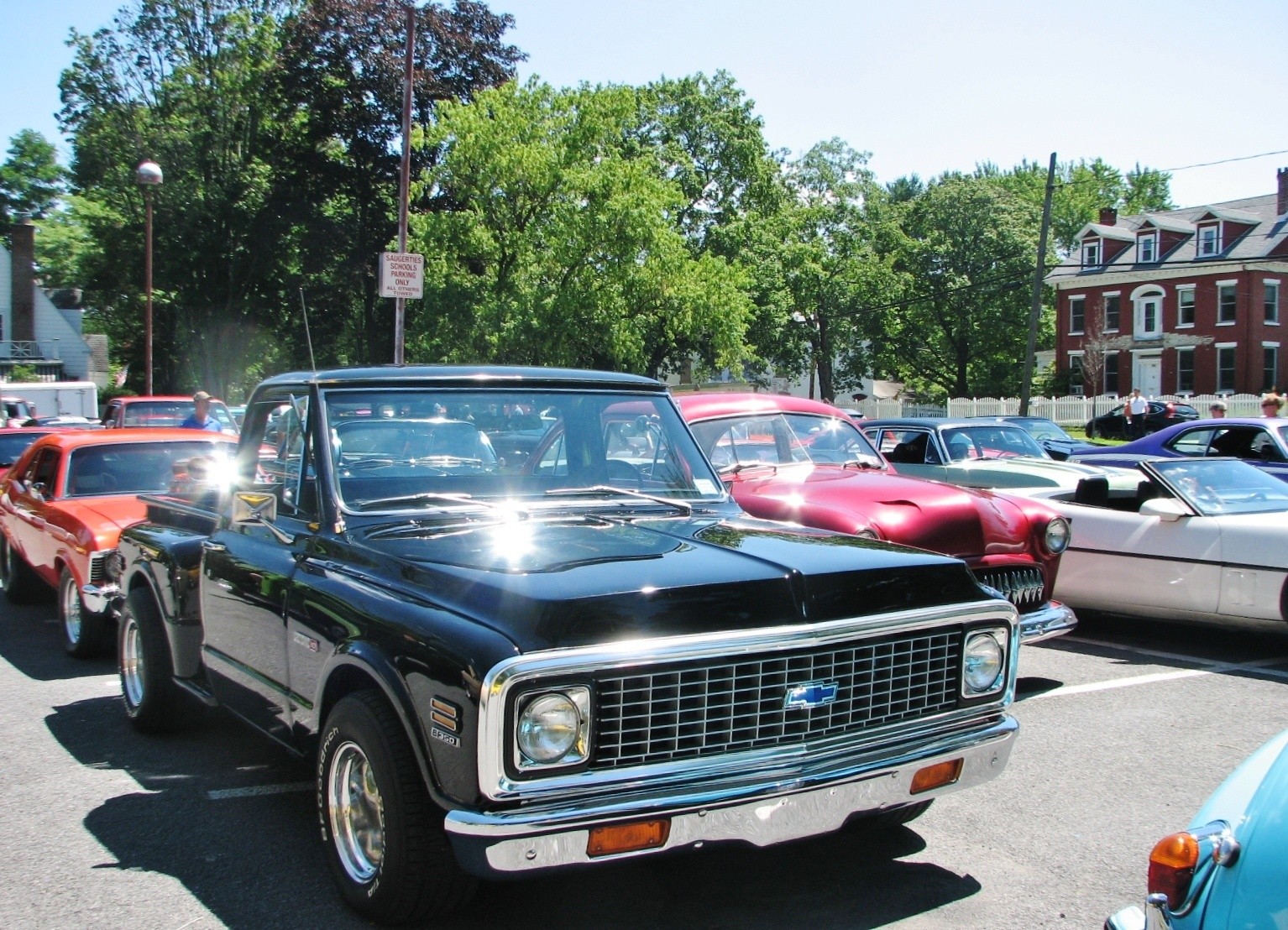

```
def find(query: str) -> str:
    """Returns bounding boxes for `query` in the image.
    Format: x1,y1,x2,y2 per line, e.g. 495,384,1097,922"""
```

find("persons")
1208,401,1229,442
490,404,524,416
1124,388,1149,441
181,391,222,433
1253,397,1284,452
433,402,474,421
277,396,315,458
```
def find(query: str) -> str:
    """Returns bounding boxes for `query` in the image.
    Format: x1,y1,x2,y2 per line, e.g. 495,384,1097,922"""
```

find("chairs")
1137,481,1160,512
1073,476,1109,508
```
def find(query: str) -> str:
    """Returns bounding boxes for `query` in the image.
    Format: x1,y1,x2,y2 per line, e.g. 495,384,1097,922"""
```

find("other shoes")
1128,438,1135,441
1125,438,1128,441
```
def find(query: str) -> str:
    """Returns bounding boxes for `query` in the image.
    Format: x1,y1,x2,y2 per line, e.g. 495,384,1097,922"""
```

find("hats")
1209,401,1228,410
193,392,214,401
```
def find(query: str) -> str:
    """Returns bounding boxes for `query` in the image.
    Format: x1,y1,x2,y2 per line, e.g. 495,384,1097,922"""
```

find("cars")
0,425,281,658
563,388,1082,648
1100,727,1287,930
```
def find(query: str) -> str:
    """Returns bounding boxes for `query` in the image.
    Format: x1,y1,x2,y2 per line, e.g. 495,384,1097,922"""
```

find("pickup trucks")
0,371,1288,658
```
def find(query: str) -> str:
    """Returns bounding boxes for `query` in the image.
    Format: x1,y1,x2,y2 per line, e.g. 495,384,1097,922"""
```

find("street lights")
136,160,162,399
